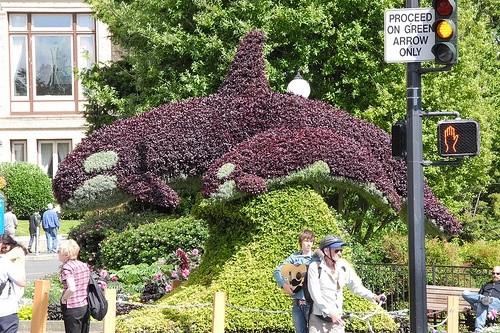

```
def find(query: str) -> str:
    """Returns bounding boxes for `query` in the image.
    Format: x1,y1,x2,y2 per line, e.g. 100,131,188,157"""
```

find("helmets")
320,236,341,249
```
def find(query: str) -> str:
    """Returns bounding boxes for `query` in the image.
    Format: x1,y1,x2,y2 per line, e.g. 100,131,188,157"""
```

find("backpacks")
303,257,319,305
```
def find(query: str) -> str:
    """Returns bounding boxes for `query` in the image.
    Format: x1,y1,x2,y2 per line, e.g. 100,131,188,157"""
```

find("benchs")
426,285,500,333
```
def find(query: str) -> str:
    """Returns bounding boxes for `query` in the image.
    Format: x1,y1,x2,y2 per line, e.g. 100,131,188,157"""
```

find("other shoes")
481,297,493,305
50,249,56,253
28,248,32,253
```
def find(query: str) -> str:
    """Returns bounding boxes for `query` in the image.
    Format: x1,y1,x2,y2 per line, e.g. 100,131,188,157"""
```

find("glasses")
492,272,500,275
327,249,342,254
1,245,8,249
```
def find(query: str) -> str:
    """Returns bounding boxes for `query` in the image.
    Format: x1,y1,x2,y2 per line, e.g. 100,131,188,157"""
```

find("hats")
326,242,347,248
47,203,53,210
35,209,40,212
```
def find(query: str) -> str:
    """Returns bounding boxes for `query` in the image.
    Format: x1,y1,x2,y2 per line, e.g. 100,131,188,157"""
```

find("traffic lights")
430,0,458,67
436,120,482,156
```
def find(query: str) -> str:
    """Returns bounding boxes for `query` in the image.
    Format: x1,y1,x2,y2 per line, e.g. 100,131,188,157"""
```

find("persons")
4,206,18,239
307,236,387,333
0,234,28,333
57,240,90,333
462,265,500,333
41,203,60,254
274,229,324,333
27,208,41,256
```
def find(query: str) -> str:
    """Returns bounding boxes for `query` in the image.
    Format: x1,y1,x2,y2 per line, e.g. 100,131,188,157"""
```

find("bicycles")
340,290,387,333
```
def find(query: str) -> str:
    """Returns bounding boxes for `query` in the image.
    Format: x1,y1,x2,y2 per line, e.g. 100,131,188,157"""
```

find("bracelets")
63,296,68,301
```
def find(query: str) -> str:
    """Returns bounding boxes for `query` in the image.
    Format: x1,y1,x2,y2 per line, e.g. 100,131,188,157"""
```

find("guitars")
281,263,308,295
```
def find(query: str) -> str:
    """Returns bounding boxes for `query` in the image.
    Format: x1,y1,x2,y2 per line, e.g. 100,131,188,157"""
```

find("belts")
296,300,307,306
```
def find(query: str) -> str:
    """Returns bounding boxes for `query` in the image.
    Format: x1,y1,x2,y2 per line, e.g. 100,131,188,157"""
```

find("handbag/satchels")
87,273,108,321
316,315,333,322
